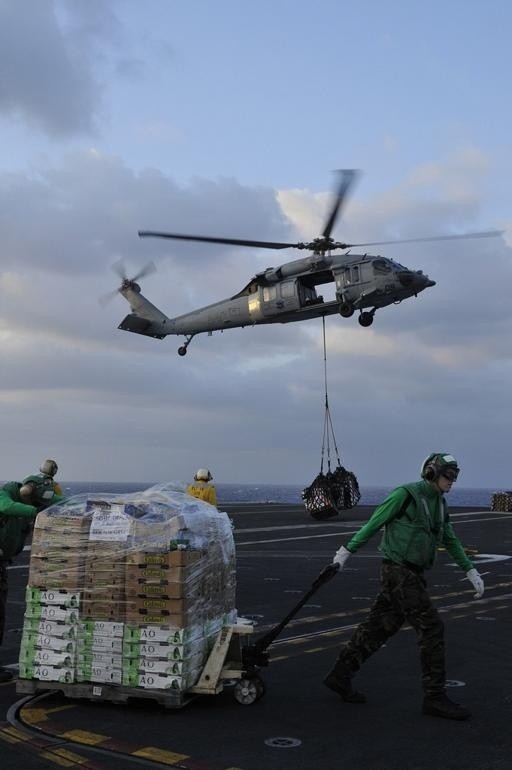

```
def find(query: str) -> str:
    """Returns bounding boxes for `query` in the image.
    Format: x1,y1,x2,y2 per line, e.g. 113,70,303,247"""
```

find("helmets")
420,452,458,478
22,475,53,507
194,468,213,481
40,460,58,477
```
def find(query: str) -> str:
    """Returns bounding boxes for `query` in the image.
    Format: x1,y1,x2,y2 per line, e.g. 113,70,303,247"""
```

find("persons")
322,453,484,720
36,459,63,497
188,469,217,507
0,474,81,684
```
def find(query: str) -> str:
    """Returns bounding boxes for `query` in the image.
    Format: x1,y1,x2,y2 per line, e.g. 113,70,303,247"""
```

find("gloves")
332,544,352,573
465,568,485,600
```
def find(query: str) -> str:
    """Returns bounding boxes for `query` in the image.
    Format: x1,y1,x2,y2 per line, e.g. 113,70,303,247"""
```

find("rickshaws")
15,562,340,714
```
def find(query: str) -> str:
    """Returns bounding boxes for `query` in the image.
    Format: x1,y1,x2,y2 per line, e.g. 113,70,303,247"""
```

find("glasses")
438,468,457,483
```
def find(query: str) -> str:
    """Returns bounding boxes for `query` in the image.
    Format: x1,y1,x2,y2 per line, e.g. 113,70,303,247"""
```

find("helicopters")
96,167,506,358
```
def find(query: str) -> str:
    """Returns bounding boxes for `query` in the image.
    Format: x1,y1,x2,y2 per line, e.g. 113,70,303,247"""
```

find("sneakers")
322,667,368,704
420,691,473,722
0,671,14,684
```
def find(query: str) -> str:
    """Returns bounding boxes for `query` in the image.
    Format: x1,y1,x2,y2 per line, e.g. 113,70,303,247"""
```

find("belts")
381,557,400,567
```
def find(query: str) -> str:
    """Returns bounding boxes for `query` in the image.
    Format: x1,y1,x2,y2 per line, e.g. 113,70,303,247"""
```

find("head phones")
193,471,213,481
20,485,32,497
421,456,440,482
51,461,58,475
